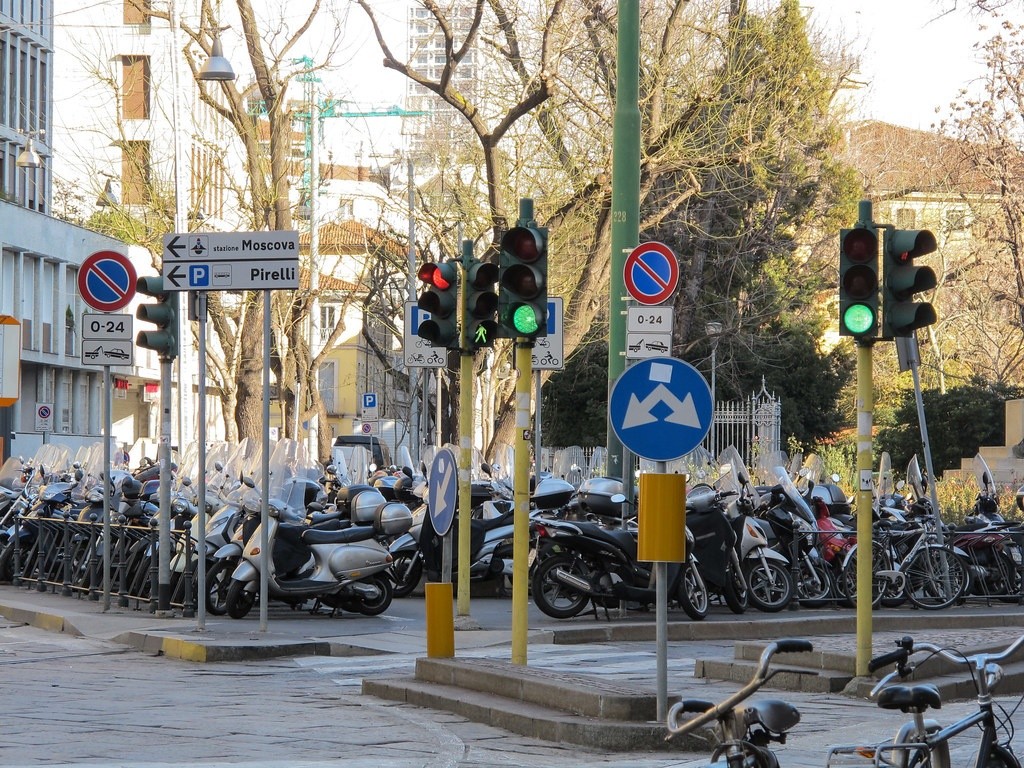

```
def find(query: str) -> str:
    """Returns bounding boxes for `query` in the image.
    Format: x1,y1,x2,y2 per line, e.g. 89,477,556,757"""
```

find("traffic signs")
158,231,300,293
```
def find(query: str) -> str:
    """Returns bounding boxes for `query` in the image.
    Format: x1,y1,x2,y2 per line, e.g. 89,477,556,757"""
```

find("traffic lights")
465,260,499,347
135,276,177,357
838,228,878,340
497,224,548,339
882,219,938,345
416,260,461,350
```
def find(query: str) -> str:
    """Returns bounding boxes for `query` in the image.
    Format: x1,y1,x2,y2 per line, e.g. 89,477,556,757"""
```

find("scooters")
1,440,412,616
411,437,1022,617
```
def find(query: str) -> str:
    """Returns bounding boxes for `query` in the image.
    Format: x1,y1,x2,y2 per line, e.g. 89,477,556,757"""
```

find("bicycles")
660,641,816,768
826,636,1024,768
